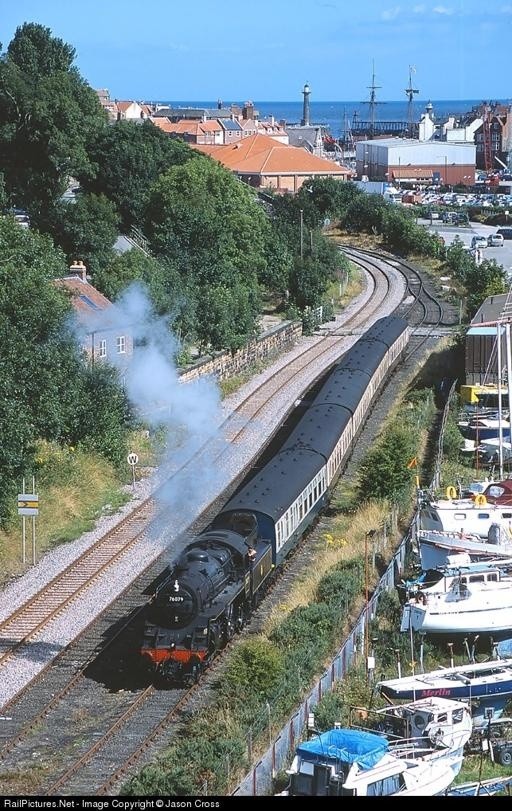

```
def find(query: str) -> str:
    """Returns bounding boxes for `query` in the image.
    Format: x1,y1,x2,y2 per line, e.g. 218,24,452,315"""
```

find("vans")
497,229,512,239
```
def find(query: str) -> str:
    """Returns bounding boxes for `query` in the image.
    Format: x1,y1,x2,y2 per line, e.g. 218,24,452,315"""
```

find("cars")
471,235,489,249
478,169,512,181
430,210,469,227
492,234,504,248
401,189,511,208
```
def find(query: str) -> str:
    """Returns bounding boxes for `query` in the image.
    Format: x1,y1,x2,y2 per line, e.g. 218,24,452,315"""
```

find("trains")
138,316,409,690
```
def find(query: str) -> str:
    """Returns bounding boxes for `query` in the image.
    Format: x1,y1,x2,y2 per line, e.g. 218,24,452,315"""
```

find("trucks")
348,180,401,204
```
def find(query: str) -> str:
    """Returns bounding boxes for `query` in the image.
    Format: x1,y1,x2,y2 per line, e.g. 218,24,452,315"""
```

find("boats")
275,659,512,796
458,404,511,469
398,473,512,640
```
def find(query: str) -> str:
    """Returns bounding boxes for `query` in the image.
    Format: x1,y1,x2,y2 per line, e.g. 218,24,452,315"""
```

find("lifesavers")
447,486,456,499
475,494,487,506
